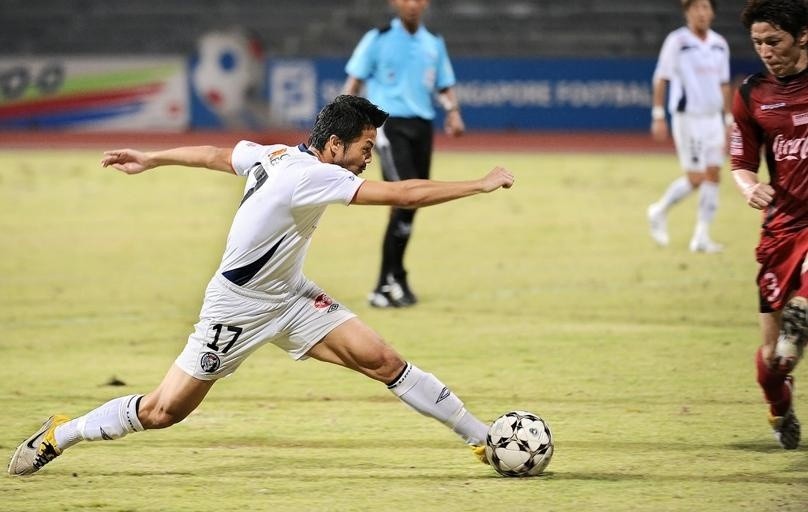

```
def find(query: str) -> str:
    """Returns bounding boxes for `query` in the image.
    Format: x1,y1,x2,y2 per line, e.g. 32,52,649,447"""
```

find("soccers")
485,409,553,477
188,21,264,118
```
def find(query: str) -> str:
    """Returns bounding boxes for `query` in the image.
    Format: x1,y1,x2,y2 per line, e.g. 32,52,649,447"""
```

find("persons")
343,1,466,309
729,1,807,450
645,1,733,253
6,96,514,475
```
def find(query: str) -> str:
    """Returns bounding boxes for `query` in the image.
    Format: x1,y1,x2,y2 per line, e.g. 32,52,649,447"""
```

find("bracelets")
651,106,666,119
724,113,735,126
446,106,462,112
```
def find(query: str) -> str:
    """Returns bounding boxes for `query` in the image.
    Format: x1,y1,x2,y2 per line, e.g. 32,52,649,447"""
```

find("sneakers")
8,415,71,475
370,271,414,307
770,295,808,372
647,206,669,243
767,377,800,449
688,236,721,252
